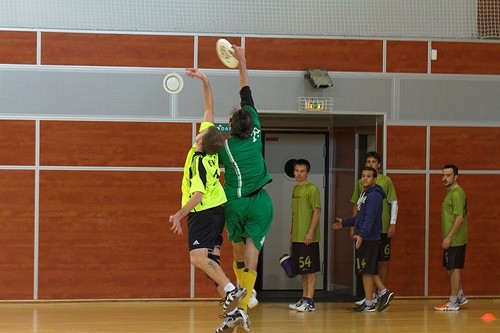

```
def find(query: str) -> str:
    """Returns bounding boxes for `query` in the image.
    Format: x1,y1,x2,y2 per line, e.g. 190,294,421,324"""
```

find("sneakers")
434,293,469,311
377,289,394,311
248,289,258,308
215,307,251,333
354,293,379,305
351,300,376,311
288,298,316,312
219,288,248,316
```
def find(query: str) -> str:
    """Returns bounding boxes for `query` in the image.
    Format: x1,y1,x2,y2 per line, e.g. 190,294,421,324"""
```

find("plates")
163,73,184,95
216,39,240,69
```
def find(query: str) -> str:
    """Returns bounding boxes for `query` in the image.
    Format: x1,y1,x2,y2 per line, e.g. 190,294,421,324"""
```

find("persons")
287,159,321,313
332,167,394,312
434,164,469,312
218,44,274,333
350,152,398,305
169,68,247,333
